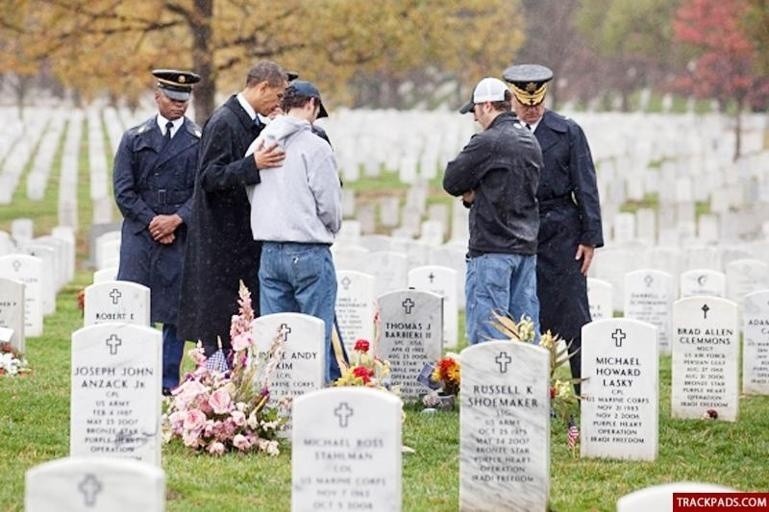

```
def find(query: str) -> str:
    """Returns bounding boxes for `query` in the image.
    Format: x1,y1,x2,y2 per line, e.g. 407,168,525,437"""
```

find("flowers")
432,355,463,396
483,310,588,411
165,280,290,458
329,337,407,430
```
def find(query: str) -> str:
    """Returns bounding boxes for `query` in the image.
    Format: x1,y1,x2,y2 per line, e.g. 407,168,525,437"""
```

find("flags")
566,417,578,449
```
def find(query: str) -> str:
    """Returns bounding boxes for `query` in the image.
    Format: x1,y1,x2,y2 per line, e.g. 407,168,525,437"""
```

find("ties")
164,123,173,143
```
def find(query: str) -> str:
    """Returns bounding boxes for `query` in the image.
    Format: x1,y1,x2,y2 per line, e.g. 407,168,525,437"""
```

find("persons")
501,66,605,405
194,62,350,385
115,69,202,394
440,77,544,348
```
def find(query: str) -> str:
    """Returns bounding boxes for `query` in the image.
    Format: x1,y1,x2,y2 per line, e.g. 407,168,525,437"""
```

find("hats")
284,80,328,118
152,69,201,101
459,65,553,114
